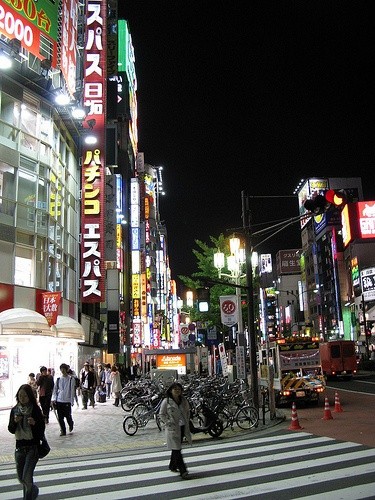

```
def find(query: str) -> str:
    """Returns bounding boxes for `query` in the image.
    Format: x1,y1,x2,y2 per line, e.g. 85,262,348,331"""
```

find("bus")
319,341,357,379
260,337,328,408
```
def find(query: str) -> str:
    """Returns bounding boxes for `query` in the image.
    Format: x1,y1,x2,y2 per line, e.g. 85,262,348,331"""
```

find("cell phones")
15,413,20,416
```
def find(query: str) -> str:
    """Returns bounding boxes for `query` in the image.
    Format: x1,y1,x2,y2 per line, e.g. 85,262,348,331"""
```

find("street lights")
214,236,261,386
175,289,209,372
274,288,319,336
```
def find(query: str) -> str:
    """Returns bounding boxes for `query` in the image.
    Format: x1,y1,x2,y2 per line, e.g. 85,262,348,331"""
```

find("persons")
27,373,38,405
34,367,54,425
67,362,136,410
158,383,195,480
7,384,51,500
53,363,76,436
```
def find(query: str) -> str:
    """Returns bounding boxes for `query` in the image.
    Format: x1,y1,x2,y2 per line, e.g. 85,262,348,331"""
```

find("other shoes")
82,403,87,410
89,401,95,407
181,472,191,480
44,418,48,424
60,431,66,436
69,425,73,432
170,467,177,472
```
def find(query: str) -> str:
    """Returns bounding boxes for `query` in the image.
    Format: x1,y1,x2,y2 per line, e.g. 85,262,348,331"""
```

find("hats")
84,362,89,365
97,364,101,367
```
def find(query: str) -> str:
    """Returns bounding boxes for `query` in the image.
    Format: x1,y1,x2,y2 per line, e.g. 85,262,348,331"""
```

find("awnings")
55,315,85,343
0,307,55,338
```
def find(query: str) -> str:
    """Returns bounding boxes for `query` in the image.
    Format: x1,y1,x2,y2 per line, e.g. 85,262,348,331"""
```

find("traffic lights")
303,188,345,215
241,296,247,311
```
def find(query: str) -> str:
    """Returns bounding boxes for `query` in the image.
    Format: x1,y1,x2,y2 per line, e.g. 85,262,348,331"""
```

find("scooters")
181,394,223,442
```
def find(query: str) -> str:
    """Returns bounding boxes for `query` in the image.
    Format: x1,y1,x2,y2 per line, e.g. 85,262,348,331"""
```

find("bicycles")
118,370,260,437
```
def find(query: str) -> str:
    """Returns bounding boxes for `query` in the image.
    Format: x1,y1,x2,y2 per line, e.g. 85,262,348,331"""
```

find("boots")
113,398,118,406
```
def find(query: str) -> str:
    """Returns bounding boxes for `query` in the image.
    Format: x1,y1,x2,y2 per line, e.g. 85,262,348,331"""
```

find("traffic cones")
321,396,336,422
286,401,304,433
332,390,344,414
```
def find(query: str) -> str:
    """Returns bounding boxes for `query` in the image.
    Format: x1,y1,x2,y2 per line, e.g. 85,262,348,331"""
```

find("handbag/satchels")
39,388,46,396
35,432,50,458
53,401,57,410
